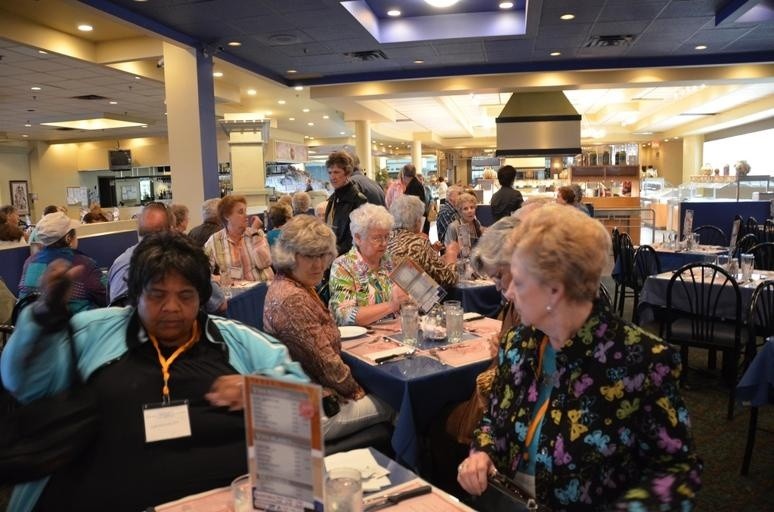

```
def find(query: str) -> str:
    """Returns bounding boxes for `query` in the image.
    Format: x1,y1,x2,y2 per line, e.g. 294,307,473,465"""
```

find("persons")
0,232,309,511
454,202,704,512
0,148,591,443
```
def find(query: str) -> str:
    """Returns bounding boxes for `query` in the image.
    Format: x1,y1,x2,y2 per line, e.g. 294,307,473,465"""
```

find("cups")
716,253,756,284
400,302,420,347
322,465,362,512
664,230,701,251
231,474,250,510
442,300,465,344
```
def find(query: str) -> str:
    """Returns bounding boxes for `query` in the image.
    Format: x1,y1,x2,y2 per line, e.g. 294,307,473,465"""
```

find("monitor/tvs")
107,149,133,170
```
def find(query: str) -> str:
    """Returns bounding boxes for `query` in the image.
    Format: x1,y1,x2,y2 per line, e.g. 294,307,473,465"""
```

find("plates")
335,325,369,339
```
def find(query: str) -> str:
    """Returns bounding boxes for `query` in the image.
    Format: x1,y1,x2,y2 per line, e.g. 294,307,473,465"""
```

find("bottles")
590,149,627,167
701,159,750,177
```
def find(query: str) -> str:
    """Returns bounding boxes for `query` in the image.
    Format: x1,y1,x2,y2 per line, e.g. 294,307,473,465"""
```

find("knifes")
361,486,435,511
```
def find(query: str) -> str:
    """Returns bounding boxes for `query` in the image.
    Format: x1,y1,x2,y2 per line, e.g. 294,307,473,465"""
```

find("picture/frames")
10,180,30,217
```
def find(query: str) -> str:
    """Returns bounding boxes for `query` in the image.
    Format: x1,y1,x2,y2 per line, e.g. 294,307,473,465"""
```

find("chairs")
0,291,41,345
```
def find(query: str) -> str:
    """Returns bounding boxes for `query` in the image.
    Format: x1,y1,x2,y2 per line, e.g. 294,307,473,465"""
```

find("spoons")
374,350,418,363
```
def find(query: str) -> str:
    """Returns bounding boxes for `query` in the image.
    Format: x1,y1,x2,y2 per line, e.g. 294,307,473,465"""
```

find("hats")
35,211,80,247
29,227,44,245
